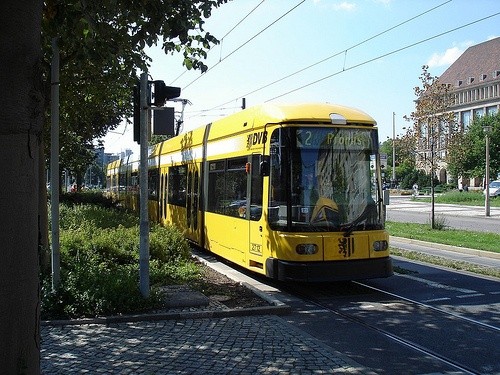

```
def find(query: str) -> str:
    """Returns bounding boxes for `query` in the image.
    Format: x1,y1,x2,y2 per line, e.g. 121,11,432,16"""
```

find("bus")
107,102,394,287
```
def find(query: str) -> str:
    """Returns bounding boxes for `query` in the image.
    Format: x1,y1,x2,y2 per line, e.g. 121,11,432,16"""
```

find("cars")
483,180,500,197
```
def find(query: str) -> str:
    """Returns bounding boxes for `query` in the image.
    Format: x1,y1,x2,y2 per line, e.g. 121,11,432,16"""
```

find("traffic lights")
154,80,182,106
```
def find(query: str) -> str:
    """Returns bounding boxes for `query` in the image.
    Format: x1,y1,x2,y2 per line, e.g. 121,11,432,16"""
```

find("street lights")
482,125,492,216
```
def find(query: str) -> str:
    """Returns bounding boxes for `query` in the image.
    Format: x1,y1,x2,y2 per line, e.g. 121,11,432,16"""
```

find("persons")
47,185,97,193
305,174,314,189
458,182,463,192
413,182,418,196
464,183,468,192
